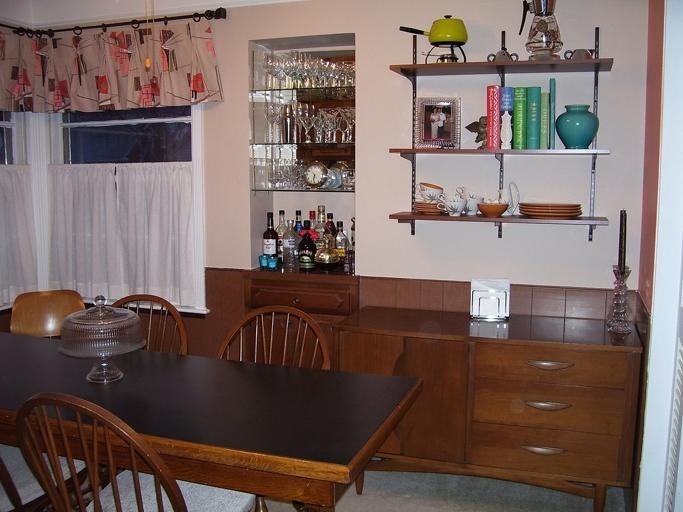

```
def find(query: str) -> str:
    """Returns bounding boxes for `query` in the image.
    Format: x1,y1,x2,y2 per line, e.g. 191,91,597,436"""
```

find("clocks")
303,160,328,188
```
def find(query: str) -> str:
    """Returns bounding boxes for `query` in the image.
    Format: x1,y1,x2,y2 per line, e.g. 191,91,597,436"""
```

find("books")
485,77,556,150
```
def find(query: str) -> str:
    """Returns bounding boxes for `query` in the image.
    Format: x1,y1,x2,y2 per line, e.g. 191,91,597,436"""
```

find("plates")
414,182,584,220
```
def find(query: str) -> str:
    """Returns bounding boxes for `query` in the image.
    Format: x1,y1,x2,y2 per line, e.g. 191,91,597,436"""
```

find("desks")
0,327,422,511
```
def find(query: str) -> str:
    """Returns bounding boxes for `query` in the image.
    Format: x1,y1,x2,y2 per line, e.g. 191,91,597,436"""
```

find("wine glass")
253,49,354,147
253,149,297,191
56,293,146,384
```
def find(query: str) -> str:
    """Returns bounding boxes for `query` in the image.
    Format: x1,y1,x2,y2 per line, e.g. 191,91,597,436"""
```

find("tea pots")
520,1,563,63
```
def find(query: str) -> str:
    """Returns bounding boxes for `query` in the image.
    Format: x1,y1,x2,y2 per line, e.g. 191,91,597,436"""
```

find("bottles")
256,202,354,275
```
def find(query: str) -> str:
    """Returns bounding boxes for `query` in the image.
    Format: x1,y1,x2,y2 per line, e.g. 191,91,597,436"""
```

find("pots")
398,14,472,49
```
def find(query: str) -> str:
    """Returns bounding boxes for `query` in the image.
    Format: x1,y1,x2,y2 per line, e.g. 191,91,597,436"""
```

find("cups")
340,170,354,192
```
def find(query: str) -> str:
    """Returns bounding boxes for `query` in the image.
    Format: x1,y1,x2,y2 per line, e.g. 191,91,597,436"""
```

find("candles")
615,208,629,270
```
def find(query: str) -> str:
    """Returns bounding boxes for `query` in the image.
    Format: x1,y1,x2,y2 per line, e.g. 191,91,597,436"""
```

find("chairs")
8,290,85,340
13,391,270,512
0,443,87,512
108,291,189,354
213,301,333,371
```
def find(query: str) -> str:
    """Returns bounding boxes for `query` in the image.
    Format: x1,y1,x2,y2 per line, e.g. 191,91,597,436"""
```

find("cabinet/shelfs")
244,270,360,370
389,25,611,243
469,336,638,507
333,325,466,474
245,40,354,273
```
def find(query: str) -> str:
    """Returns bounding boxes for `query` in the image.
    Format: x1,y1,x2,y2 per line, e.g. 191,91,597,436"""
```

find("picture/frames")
412,95,461,150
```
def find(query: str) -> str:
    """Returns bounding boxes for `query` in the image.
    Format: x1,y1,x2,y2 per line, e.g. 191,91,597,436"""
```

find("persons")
428,107,439,139
437,108,446,139
